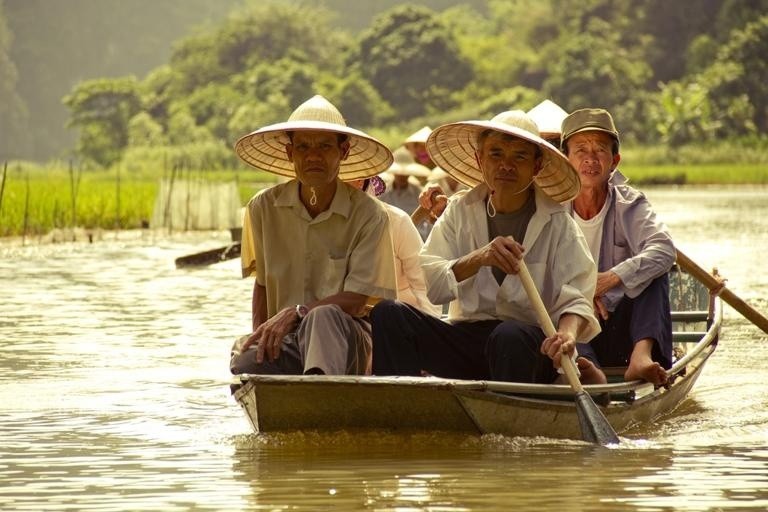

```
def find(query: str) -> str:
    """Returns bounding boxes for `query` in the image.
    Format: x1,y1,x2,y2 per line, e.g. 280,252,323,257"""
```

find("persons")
345,100,571,318
370,109,602,384
230,93,397,375
552,109,679,385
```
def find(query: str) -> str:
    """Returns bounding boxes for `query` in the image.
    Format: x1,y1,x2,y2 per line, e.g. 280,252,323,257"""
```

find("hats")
370,126,449,195
233,94,394,183
526,100,619,149
425,109,581,204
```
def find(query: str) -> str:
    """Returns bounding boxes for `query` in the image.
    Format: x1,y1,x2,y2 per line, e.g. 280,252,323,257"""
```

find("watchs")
295,303,310,319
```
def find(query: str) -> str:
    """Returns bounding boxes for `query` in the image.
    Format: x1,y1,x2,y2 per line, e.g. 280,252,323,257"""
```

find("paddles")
176,245,240,268
506,235,619,445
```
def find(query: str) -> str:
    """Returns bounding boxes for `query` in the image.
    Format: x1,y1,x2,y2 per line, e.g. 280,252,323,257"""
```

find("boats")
230,266,723,433
